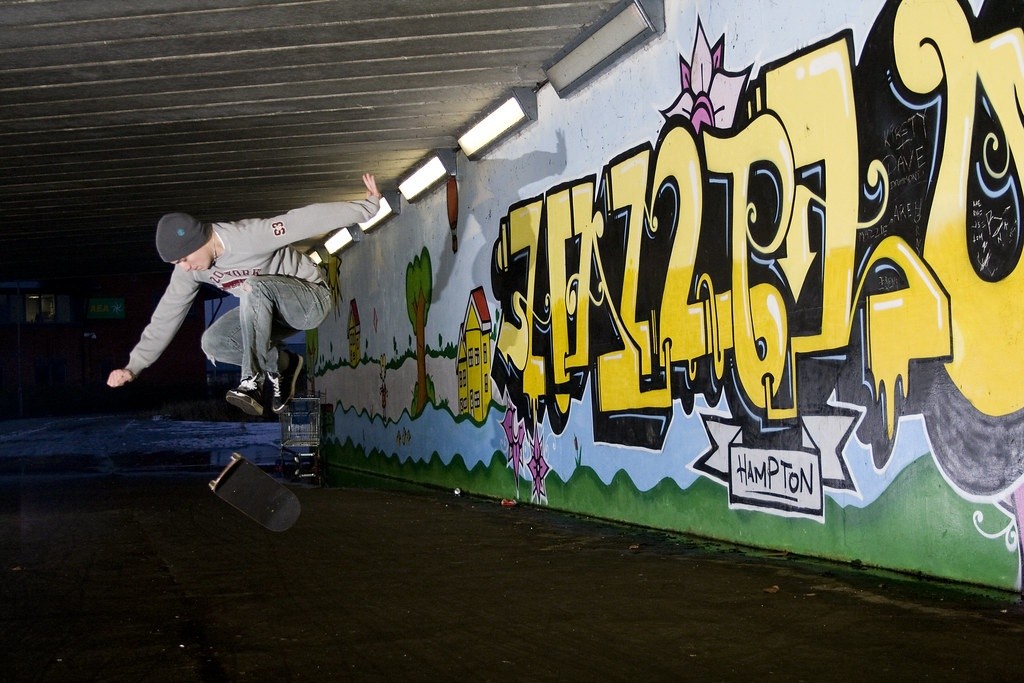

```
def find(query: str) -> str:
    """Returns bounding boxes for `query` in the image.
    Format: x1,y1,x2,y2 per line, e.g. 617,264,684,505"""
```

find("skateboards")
208,450,302,535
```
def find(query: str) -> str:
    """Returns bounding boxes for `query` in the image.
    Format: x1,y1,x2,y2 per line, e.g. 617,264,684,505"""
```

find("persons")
106,173,384,416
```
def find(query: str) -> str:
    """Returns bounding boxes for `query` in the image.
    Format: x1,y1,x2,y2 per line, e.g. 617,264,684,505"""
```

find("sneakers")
267,348,303,413
226,372,264,415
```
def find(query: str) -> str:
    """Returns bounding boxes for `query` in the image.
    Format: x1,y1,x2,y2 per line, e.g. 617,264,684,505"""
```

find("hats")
155,213,209,262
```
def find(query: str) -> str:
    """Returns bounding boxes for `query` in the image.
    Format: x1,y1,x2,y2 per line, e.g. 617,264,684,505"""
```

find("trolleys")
277,395,324,486
